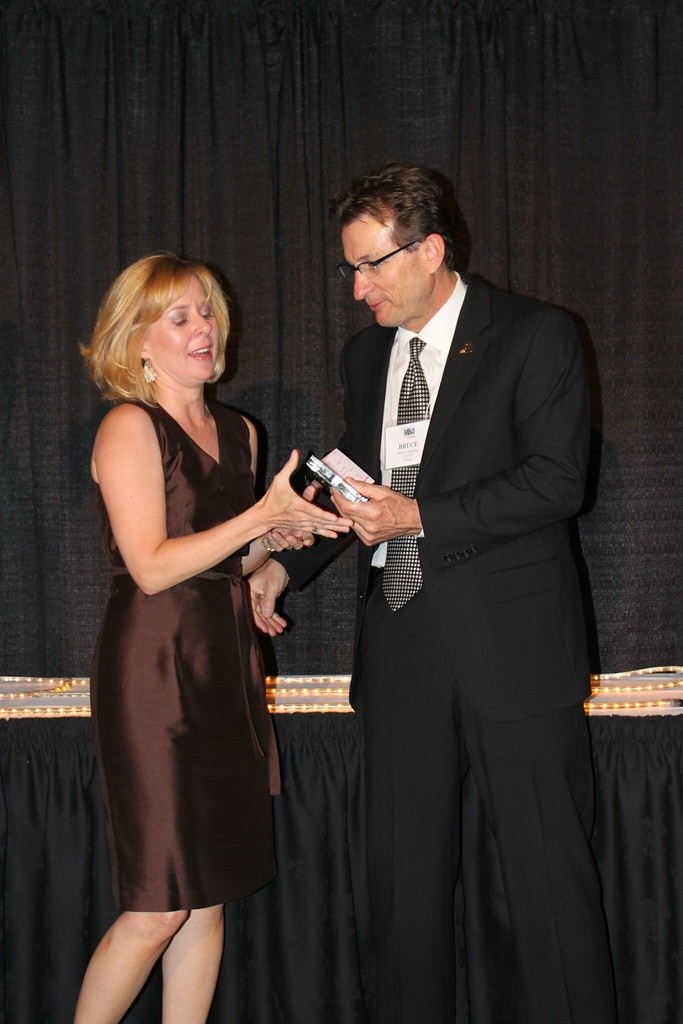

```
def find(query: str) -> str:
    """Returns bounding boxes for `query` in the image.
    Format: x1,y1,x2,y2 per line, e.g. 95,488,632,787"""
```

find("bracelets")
261,535,277,552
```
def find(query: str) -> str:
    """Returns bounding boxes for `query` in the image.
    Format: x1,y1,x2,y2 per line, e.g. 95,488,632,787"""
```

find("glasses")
338,234,423,281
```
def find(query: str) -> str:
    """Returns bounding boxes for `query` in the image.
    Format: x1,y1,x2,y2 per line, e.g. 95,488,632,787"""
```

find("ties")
382,336,429,611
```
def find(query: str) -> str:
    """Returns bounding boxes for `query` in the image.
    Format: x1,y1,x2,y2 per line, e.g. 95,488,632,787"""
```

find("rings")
311,524,319,533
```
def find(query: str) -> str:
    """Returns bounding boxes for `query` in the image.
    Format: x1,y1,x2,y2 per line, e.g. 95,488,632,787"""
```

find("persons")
247,164,624,1024
71,255,354,1024
